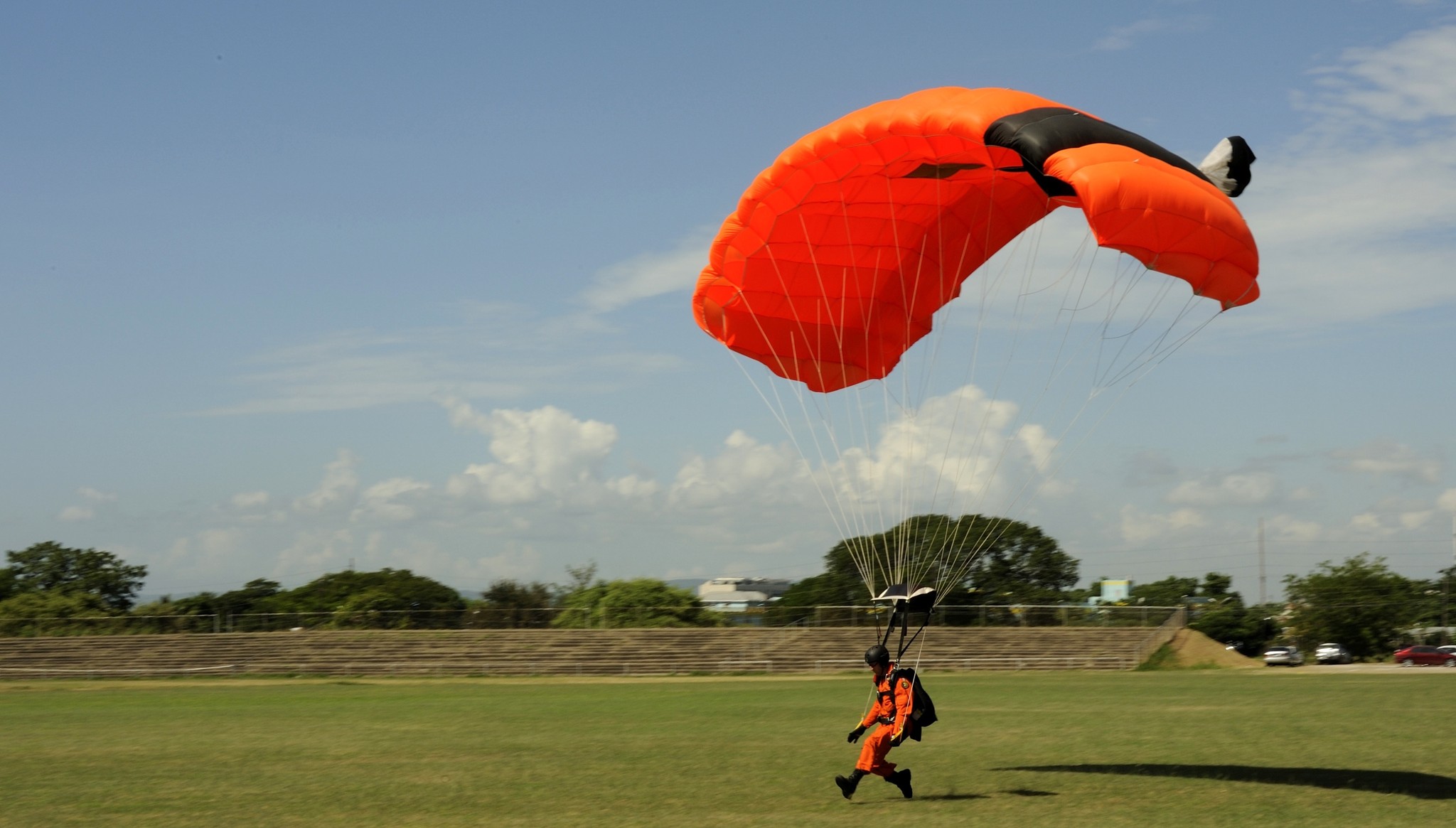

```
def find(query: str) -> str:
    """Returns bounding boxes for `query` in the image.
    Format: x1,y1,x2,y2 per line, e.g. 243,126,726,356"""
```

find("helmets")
865,645,890,667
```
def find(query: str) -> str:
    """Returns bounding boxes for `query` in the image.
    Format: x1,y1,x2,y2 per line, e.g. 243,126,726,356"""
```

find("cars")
1394,644,1456,667
1315,643,1353,664
1262,646,1306,665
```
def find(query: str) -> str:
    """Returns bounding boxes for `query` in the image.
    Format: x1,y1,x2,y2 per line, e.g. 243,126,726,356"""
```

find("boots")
835,768,864,799
884,769,912,798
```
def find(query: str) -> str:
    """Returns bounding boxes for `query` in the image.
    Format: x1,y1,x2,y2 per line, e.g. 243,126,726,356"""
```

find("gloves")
888,734,900,747
847,724,867,743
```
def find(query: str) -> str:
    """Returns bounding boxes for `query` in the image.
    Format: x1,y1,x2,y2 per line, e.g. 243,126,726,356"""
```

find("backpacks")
889,667,937,727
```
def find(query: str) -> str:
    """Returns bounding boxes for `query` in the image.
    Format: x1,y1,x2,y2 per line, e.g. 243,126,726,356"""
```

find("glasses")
869,662,877,666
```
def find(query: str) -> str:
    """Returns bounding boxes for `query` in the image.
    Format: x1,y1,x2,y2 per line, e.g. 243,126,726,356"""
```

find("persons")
834,645,914,799
